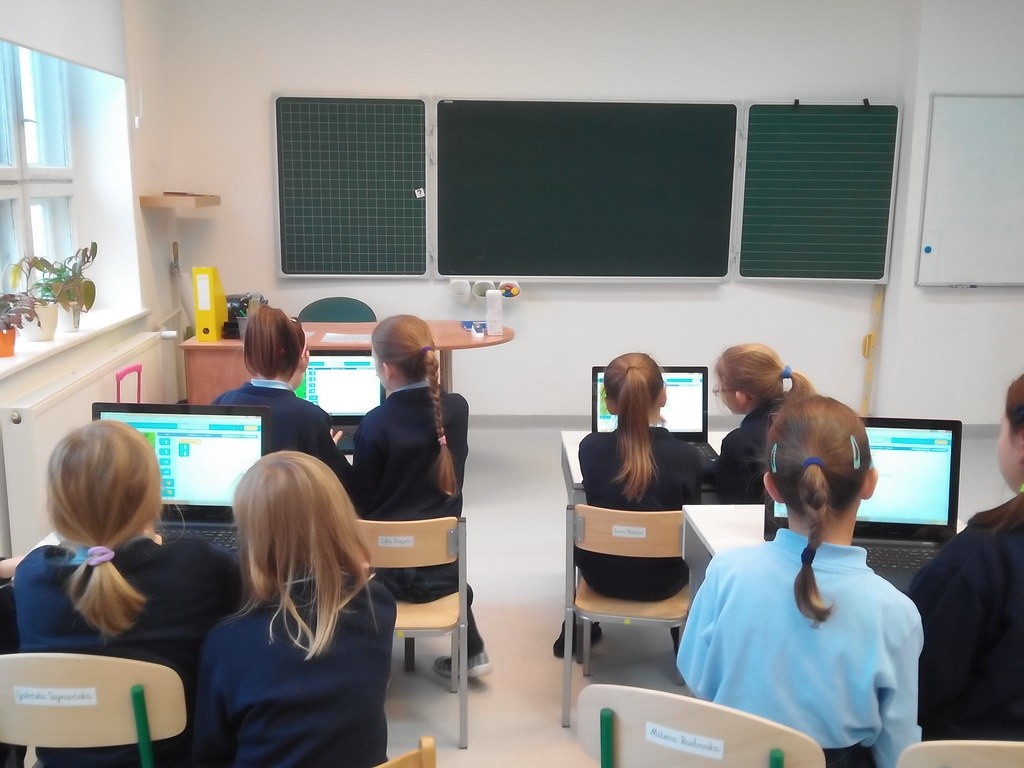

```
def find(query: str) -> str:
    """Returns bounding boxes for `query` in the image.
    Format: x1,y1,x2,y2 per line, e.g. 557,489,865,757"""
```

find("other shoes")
435,651,492,677
553,620,602,658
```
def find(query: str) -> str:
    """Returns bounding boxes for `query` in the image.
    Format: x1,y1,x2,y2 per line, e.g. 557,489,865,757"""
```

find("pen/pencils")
230,303,246,317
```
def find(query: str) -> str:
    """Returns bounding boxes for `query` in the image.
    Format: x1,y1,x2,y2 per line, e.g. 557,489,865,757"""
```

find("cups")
236,316,247,342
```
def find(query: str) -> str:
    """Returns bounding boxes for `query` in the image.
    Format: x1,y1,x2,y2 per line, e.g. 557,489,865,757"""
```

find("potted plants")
8,254,60,341
0,293,41,358
42,240,99,334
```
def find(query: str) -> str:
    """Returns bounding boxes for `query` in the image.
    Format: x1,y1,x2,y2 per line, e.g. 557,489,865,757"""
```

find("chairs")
0,651,186,768
575,682,827,768
297,297,377,357
561,504,691,729
352,516,470,751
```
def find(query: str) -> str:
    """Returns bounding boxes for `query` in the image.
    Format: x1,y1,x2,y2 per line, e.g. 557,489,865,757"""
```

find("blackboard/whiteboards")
437,97,736,278
915,92,1024,288
274,97,429,275
737,102,902,283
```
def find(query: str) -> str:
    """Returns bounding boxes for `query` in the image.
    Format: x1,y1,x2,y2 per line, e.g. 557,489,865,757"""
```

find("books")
461,321,486,330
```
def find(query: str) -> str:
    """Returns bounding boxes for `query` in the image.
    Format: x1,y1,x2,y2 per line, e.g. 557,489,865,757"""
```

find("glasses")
713,382,751,400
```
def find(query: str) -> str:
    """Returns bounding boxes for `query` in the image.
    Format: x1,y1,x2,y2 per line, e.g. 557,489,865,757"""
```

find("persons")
198,451,399,768
909,374,1024,740
704,345,816,503
677,393,924,768
0,557,27,768
210,304,352,494
553,352,705,659
14,420,242,768
351,314,494,678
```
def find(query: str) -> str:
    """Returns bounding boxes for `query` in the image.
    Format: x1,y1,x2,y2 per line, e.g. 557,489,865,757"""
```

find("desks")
678,504,966,596
559,430,732,505
176,321,516,406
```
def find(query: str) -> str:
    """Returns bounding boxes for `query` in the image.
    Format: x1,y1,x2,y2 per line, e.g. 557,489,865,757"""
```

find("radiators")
0,329,167,557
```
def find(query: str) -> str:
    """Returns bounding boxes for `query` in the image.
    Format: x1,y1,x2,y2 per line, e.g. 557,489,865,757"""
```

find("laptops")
764,413,963,597
591,366,720,463
92,403,271,561
294,350,387,455
